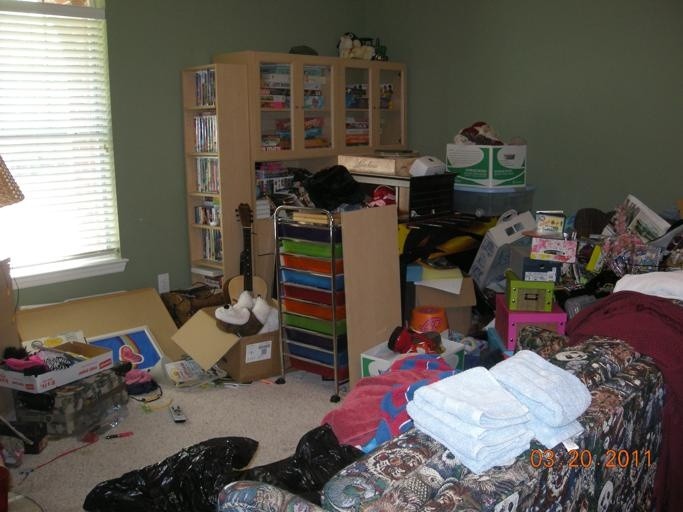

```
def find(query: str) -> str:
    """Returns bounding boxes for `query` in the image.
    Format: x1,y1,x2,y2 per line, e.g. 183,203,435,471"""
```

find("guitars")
224,202,267,306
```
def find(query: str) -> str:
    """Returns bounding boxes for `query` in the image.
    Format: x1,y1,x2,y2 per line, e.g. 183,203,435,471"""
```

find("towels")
403,347,594,474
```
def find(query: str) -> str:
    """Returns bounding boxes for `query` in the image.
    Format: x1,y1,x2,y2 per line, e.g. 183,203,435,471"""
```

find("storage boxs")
360,139,578,380
169,297,289,382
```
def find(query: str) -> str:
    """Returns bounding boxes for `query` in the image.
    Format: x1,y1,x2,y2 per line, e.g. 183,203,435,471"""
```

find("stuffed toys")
214,289,279,338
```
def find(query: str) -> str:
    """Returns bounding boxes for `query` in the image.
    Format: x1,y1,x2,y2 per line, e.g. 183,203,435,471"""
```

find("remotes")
169,404,186,423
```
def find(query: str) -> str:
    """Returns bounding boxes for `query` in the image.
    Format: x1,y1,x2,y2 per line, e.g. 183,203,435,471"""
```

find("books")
192,69,223,289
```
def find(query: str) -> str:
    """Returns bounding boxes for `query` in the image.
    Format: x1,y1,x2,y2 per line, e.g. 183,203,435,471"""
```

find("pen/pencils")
105,431,133,439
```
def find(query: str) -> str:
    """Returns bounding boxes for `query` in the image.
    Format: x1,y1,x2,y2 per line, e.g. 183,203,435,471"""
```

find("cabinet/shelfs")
180,50,461,403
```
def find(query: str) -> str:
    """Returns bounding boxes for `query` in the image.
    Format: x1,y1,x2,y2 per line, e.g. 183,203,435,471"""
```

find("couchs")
216,333,666,511
